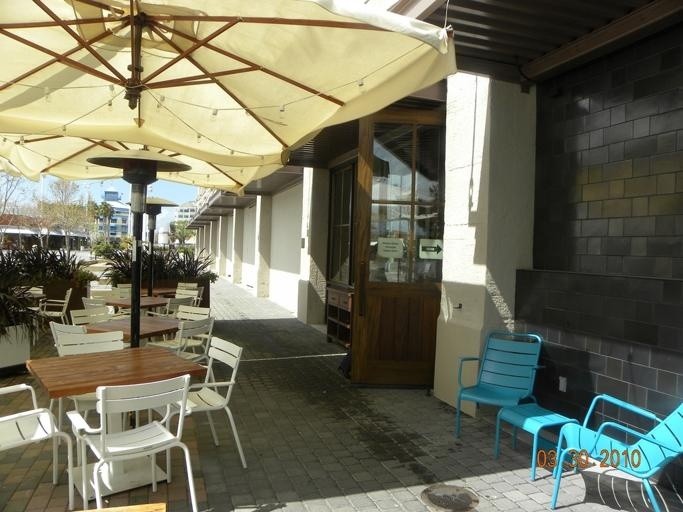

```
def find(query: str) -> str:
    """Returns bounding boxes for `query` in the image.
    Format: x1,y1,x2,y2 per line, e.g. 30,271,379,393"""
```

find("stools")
494,403,579,481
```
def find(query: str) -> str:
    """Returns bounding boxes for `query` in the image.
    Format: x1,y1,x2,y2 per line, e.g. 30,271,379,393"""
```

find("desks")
13,286,46,298
85,317,185,341
26,346,207,501
143,288,177,295
105,298,171,309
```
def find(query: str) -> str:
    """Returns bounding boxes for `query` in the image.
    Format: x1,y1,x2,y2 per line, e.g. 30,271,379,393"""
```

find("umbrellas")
2,0,457,166
1,130,288,197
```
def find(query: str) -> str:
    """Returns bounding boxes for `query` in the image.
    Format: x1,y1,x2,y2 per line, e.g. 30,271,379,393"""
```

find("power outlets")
456,297,464,311
559,376,567,392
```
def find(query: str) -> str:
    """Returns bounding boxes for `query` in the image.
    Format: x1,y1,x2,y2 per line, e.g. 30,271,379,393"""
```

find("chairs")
147,305,211,353
91,290,112,297
148,336,248,484
456,329,547,450
0,384,74,511
18,307,40,346
82,297,105,309
551,394,683,512
175,289,199,307
66,374,199,512
177,317,218,393
118,284,131,298
49,321,87,411
57,330,139,467
145,297,192,341
70,307,110,326
32,288,72,347
183,287,204,306
177,283,198,288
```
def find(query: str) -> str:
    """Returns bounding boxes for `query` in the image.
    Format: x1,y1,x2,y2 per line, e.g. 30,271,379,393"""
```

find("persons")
338,349,352,380
